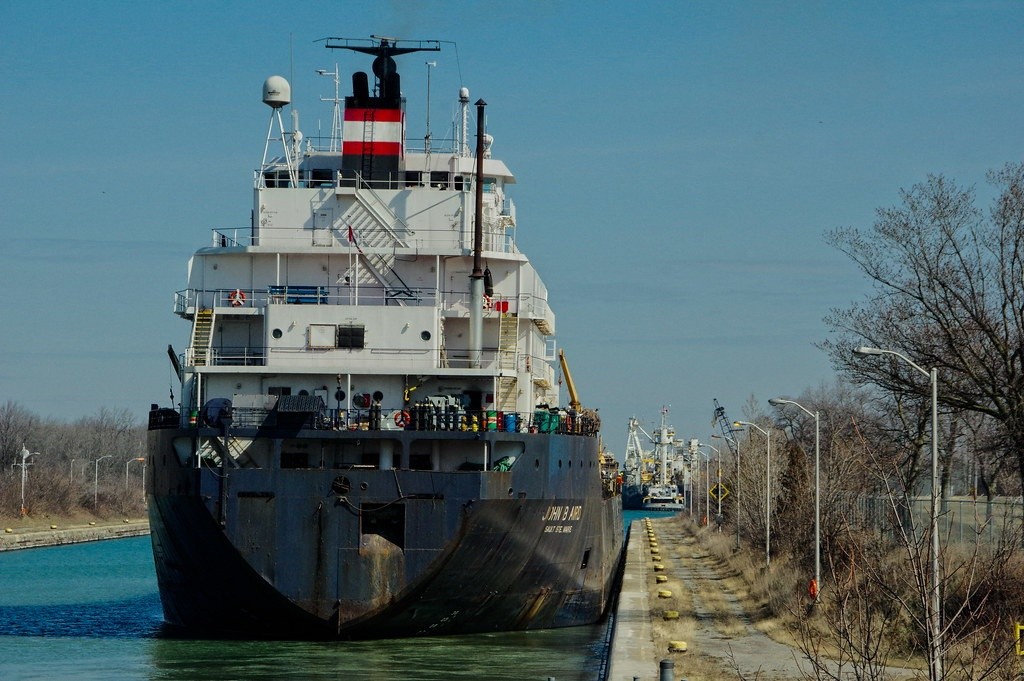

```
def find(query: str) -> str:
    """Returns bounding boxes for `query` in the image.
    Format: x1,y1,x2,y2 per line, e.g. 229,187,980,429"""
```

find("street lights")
853,345,944,680
733,420,771,570
698,443,722,535
81,461,92,486
688,449,710,530
686,452,701,519
712,434,742,550
20,451,40,519
767,397,822,605
677,454,694,518
124,457,145,495
94,455,111,513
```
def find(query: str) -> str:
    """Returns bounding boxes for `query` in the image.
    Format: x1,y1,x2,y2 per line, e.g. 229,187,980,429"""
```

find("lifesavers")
566,414,572,430
230,290,246,306
395,411,410,427
482,293,491,309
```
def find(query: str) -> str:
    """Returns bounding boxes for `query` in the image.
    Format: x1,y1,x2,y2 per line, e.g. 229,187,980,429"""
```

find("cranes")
710,397,740,468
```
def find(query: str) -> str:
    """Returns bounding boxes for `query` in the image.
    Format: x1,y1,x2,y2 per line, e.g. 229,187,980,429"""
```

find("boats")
622,401,705,512
141,29,628,634
